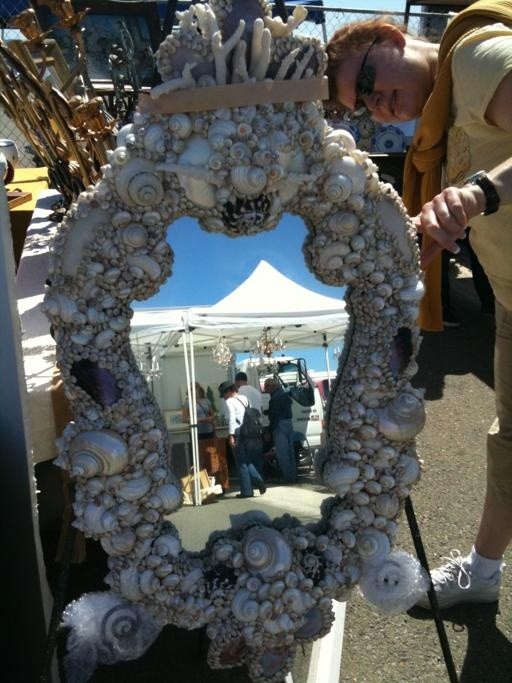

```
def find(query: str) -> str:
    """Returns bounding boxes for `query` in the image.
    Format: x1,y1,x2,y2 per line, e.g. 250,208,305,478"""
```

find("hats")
218,381,234,398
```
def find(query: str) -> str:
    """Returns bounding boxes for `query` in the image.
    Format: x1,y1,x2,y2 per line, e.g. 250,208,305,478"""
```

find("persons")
323,1,512,617
181,370,298,498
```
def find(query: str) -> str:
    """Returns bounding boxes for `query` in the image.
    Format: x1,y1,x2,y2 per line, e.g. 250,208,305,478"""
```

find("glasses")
354,35,380,117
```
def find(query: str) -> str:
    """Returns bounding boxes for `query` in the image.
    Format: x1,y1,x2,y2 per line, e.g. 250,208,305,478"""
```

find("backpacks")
234,395,265,453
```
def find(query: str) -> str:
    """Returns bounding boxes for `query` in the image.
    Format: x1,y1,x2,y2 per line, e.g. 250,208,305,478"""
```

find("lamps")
256,327,286,359
211,337,235,370
134,338,162,384
333,346,341,358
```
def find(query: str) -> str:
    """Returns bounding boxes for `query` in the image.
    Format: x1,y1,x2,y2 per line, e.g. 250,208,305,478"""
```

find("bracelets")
461,168,501,218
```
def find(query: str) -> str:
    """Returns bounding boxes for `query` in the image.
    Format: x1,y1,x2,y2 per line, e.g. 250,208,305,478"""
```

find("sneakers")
258,481,266,494
236,493,254,498
414,554,502,610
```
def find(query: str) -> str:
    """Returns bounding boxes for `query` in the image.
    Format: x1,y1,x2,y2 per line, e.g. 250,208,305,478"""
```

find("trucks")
134,348,340,464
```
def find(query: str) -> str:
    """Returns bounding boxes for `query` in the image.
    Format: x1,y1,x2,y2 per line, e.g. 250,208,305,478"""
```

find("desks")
3,167,51,275
367,153,407,199
0,188,346,681
88,77,151,131
168,425,229,475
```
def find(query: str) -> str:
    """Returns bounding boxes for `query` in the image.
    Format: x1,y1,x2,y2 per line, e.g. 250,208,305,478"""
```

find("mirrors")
40,0,427,683
